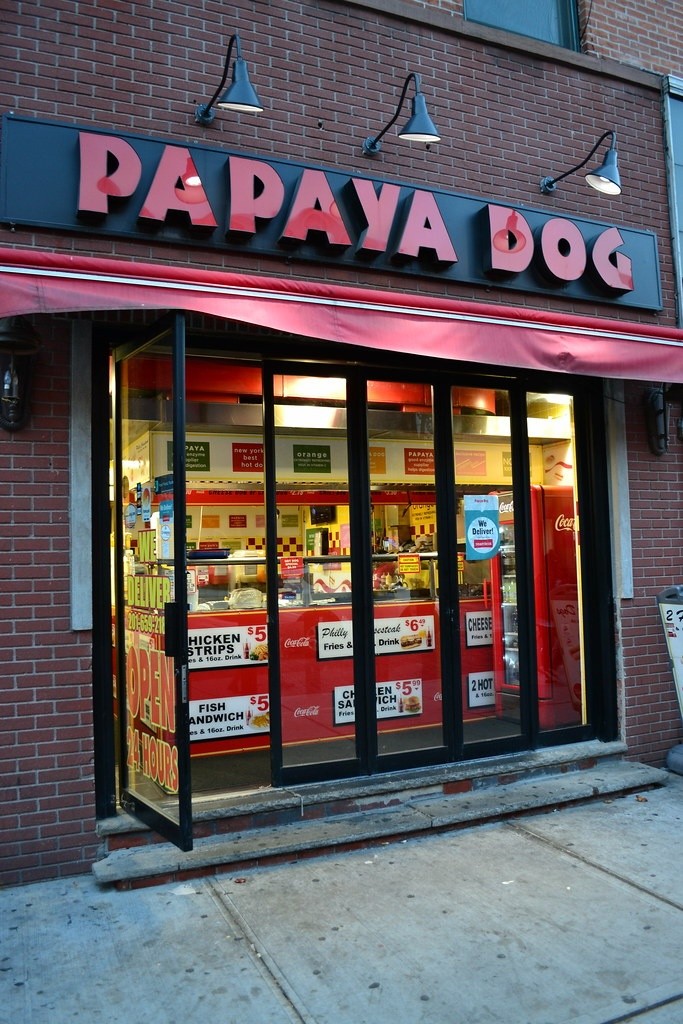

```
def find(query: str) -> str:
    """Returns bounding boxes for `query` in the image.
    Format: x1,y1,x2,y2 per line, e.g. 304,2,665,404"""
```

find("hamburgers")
401,634,423,650
405,696,422,712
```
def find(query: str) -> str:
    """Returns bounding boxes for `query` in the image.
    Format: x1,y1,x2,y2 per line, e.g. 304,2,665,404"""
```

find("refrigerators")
482,485,583,730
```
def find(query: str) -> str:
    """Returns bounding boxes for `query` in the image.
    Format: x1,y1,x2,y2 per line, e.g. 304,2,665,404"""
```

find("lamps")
0,315,42,432
195,35,264,125
540,131,620,194
644,382,683,456
361,73,442,156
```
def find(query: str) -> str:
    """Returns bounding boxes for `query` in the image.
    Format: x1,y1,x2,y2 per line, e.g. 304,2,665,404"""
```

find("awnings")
0,247,683,382
